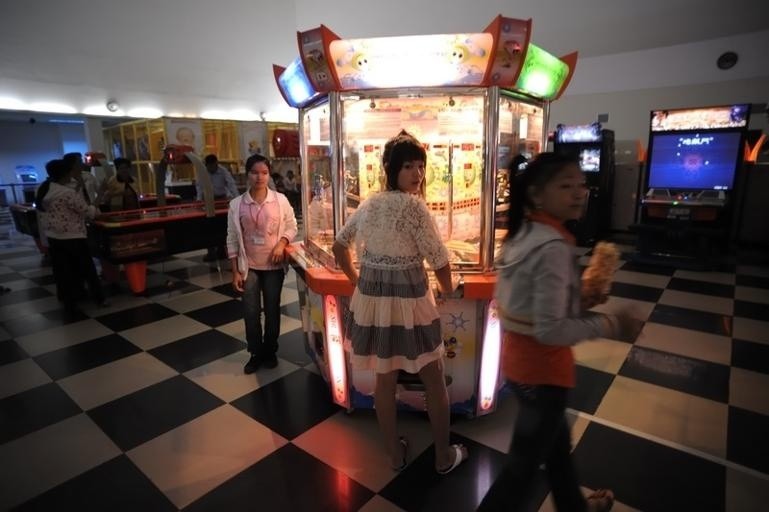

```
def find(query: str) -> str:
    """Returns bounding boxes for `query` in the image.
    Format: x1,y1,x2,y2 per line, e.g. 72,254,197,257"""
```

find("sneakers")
241,348,280,374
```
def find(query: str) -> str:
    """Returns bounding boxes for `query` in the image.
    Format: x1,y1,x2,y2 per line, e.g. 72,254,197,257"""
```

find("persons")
35,148,142,325
477,152,646,512
332,130,470,476
197,154,240,261
0,285,12,296
225,155,298,374
268,169,301,205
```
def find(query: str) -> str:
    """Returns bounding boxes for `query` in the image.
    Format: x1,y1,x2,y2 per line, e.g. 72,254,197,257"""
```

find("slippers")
386,434,412,471
433,441,468,476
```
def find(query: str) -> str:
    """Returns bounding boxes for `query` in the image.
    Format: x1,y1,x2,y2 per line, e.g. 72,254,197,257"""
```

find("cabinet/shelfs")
102,118,169,195
200,119,241,163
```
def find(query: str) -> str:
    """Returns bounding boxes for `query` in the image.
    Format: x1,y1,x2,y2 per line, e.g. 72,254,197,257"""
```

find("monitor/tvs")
21,173,37,183
648,132,741,190
578,146,601,173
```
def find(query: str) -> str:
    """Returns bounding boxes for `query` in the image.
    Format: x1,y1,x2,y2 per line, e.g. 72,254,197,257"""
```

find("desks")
10,193,231,293
